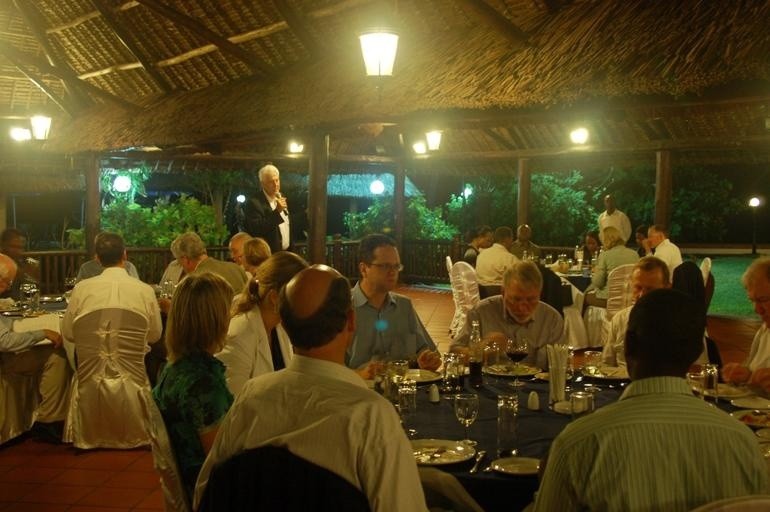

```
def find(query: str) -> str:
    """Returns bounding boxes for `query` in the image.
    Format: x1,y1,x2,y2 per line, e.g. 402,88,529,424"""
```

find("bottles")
468,320,484,386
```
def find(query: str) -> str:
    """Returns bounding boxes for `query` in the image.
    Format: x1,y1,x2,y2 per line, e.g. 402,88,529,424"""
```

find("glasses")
364,262,404,273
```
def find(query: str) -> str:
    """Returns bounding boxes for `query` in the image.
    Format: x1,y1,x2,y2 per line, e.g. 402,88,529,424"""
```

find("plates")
535,372,549,379
719,383,770,437
410,363,542,475
28,300,53,302
0,305,20,312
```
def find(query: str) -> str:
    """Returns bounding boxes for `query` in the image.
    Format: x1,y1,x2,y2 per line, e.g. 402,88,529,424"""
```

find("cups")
163,280,177,300
386,360,419,436
63,277,77,291
442,342,501,399
583,350,602,374
566,346,575,390
545,254,566,265
30,290,40,310
577,259,598,275
690,365,718,407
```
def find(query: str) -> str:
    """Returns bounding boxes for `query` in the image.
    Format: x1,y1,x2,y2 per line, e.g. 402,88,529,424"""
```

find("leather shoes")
33,421,63,444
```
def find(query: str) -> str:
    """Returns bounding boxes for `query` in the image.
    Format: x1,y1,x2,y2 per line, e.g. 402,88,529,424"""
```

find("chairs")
62,306,153,451
701,257,712,285
688,494,770,511
585,263,635,348
136,384,191,512
446,256,452,272
195,447,373,512
451,261,481,337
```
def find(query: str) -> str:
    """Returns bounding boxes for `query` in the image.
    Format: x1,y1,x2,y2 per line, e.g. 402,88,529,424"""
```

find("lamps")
30,70,55,142
355,1,402,99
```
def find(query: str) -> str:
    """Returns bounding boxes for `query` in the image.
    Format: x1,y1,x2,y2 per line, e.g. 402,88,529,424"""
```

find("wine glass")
575,249,584,266
24,283,36,302
571,392,593,418
504,337,529,386
524,250,534,261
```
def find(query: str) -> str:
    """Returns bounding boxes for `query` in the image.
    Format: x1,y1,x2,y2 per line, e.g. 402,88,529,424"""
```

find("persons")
532,288,770,511
1,236,442,511
241,165,294,254
580,193,683,296
449,261,568,367
465,226,543,286
601,255,674,380
723,256,769,394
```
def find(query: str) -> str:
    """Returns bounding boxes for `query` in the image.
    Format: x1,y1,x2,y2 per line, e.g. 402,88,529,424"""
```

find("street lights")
748,193,760,257
